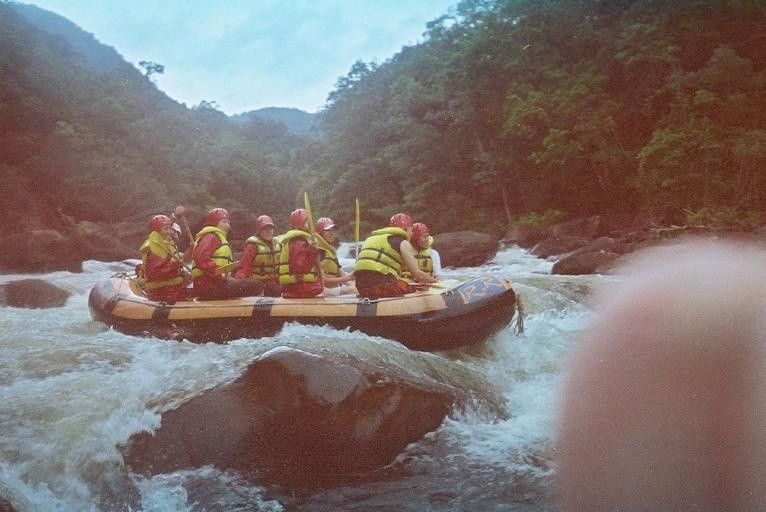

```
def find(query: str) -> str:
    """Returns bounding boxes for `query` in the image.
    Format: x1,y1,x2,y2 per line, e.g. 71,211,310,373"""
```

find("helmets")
410,222,432,240
208,208,231,226
289,208,308,227
150,214,173,232
170,222,182,240
255,215,275,236
389,213,413,230
314,218,337,234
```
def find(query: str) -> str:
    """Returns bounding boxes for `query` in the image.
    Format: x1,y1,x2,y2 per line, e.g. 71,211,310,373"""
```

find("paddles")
407,283,448,290
304,192,327,300
355,198,359,263
216,261,241,274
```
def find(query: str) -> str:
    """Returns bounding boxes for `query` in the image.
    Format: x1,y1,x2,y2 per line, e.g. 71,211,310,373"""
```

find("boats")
88,273,519,352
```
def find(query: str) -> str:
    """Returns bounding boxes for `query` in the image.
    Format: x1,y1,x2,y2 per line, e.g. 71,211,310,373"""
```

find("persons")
278,207,327,300
314,216,355,296
141,214,198,302
189,208,265,300
234,213,281,298
168,204,195,262
354,213,440,297
398,220,442,284
